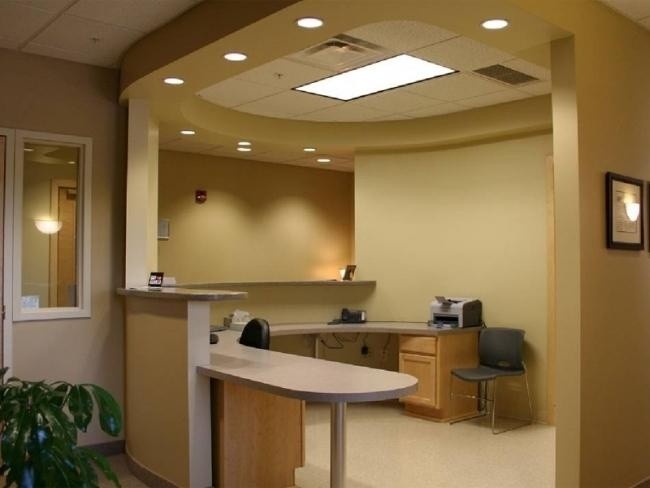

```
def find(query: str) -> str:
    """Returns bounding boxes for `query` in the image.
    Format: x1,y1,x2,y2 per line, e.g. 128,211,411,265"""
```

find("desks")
202,320,488,488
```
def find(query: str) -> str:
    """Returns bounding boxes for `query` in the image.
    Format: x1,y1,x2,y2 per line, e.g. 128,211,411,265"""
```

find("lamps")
35,221,62,234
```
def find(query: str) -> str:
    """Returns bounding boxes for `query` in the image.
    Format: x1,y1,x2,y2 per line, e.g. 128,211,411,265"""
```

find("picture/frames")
158,218,171,240
605,171,644,250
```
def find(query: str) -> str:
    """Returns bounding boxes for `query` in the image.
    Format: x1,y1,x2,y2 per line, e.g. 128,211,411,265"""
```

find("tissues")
229,309,250,331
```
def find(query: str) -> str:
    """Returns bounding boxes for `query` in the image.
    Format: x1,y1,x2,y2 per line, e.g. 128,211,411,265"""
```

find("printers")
428,296,482,328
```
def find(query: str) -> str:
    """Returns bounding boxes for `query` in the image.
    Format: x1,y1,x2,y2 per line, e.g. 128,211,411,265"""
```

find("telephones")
341,308,367,324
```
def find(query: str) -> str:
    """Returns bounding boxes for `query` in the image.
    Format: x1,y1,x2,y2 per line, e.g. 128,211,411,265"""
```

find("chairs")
450,326,534,436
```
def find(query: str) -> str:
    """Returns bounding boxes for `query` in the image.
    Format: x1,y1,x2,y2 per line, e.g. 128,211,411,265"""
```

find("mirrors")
11,128,92,322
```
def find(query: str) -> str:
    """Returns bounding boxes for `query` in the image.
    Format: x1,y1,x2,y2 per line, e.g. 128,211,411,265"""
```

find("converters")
362,346,369,354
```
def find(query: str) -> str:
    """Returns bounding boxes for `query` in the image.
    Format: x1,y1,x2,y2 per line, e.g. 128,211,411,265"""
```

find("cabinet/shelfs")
393,331,485,431
210,375,312,488
